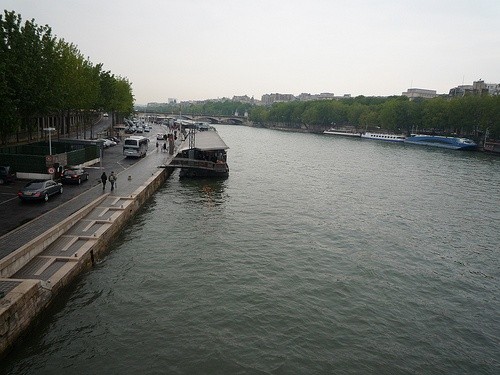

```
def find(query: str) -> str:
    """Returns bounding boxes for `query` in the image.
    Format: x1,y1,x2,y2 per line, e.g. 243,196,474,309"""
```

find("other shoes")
111,187,114,191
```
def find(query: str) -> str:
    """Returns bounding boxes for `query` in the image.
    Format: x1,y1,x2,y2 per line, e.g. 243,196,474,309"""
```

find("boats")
361,132,407,142
175,129,230,181
403,132,478,150
322,125,363,137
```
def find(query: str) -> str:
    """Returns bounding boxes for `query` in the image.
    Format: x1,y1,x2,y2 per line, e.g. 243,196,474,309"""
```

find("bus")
122,135,151,159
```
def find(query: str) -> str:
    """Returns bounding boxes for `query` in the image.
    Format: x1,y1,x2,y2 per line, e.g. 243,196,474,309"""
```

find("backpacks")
110,176,114,181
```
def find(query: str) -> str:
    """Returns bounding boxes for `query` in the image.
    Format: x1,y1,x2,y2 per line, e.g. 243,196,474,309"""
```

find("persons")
109,171,116,192
101,172,107,190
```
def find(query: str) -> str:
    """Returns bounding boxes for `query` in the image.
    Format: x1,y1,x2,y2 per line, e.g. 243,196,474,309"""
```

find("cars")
124,117,164,140
17,179,64,204
96,136,121,149
60,164,89,185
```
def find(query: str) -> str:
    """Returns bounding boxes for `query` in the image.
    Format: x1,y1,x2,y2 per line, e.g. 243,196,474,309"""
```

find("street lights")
42,127,55,180
